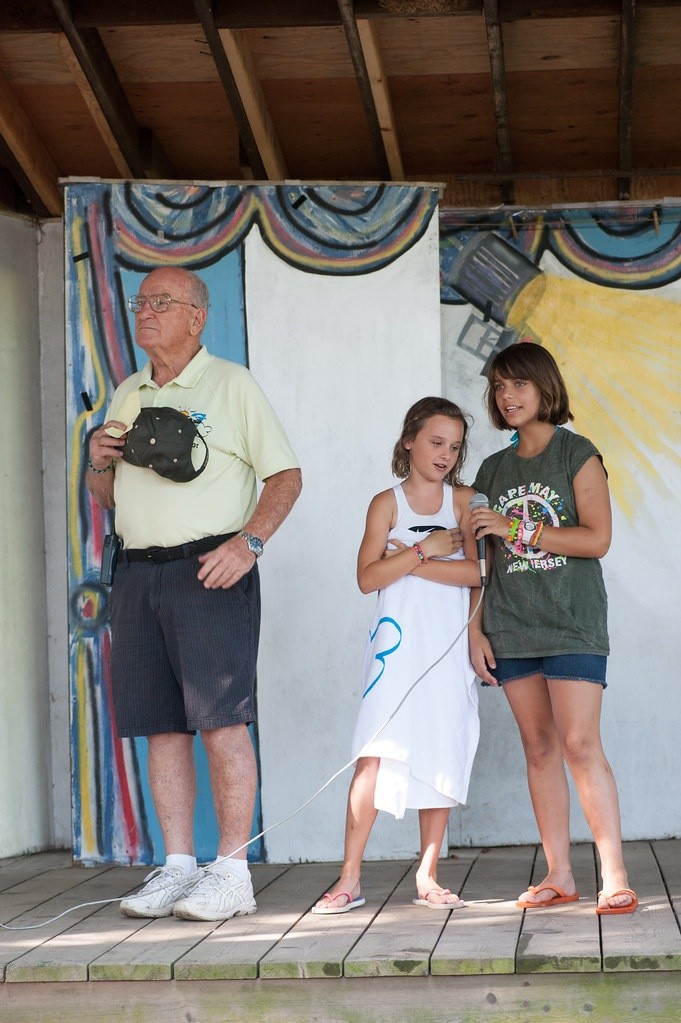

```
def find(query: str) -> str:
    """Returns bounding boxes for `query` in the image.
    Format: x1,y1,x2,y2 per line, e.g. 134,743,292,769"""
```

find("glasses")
127,294,199,313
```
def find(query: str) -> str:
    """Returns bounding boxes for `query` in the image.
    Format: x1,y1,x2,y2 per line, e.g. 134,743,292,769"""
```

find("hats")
112,407,209,483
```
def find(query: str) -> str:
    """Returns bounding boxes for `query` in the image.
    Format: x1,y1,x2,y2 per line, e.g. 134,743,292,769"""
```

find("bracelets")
529,521,544,546
507,519,525,545
412,542,428,565
88,457,115,474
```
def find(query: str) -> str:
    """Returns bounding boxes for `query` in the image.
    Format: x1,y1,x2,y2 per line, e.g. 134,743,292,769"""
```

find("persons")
310,397,480,915
471,342,638,914
86,266,303,923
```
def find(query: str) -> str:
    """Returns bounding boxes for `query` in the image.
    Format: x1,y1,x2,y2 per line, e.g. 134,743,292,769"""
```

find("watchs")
238,530,264,557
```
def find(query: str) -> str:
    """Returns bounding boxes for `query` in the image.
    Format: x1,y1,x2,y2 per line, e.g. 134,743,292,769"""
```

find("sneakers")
120,865,204,919
173,865,257,921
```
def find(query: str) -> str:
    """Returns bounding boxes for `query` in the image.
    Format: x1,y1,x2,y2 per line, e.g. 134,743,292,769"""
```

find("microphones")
469,492,489,588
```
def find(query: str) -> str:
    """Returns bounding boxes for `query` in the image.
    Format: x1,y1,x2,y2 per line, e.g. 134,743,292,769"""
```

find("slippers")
312,892,366,914
412,889,464,910
596,888,637,915
516,885,578,907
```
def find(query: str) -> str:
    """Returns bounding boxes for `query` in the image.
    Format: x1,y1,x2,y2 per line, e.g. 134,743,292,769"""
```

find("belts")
118,531,241,563
